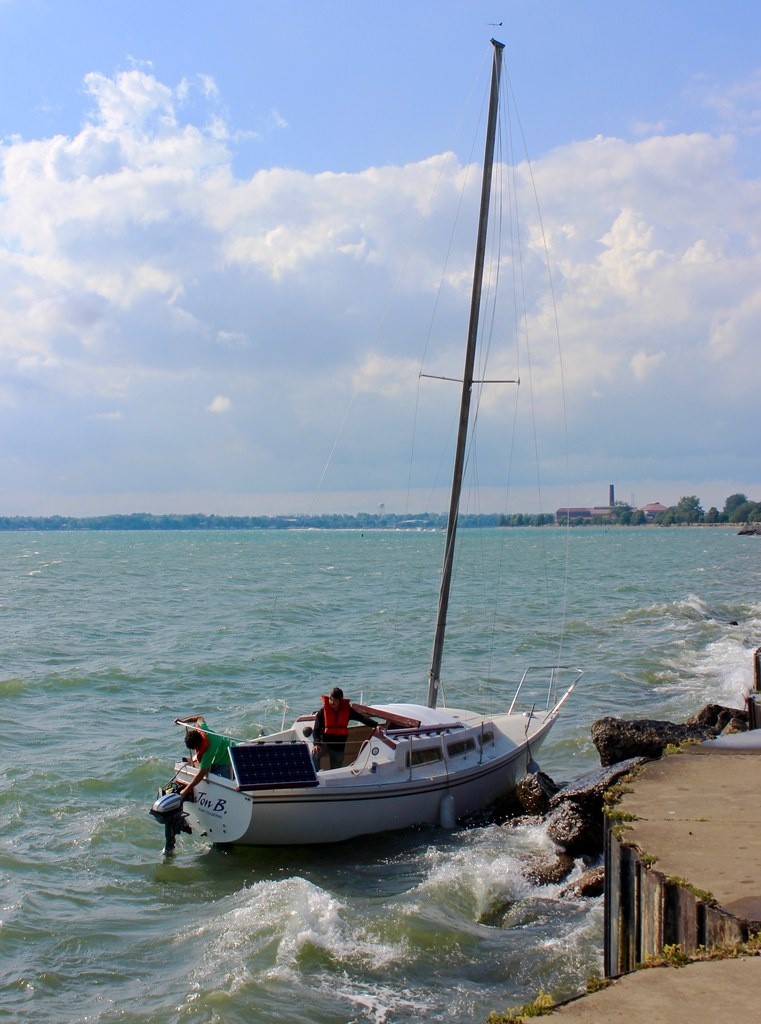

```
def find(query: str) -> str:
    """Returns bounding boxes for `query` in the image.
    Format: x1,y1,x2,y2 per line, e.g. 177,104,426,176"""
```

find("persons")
174,715,235,797
312,688,381,773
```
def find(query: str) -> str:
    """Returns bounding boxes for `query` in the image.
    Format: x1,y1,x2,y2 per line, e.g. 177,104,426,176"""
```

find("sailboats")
150,36,585,855
